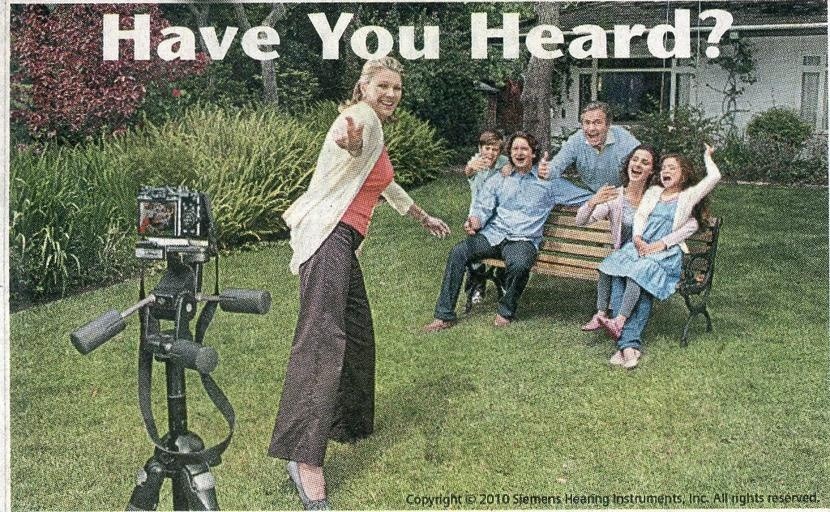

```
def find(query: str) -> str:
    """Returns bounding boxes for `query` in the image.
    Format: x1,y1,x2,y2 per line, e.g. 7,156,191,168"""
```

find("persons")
577,143,701,368
262,53,453,509
420,128,594,334
467,128,514,211
581,143,723,340
536,99,647,194
134,203,180,236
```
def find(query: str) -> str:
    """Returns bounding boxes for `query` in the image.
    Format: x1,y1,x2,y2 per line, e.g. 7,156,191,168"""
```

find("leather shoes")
424,318,458,332
608,346,642,369
286,462,330,510
596,315,621,339
581,312,608,331
494,312,513,329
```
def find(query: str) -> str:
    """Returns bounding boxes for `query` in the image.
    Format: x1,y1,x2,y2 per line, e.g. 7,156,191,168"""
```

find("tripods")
70,264,272,511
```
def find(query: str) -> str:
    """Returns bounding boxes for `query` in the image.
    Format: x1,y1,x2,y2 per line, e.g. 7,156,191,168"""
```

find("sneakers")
472,285,508,305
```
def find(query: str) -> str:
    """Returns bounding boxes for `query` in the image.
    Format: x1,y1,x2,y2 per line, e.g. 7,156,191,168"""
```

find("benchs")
465,203,722,347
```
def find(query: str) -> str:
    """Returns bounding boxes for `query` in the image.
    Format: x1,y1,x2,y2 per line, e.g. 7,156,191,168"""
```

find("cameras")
135,185,216,263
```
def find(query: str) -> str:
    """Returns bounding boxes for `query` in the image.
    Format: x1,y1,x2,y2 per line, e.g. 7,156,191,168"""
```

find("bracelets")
420,211,428,224
